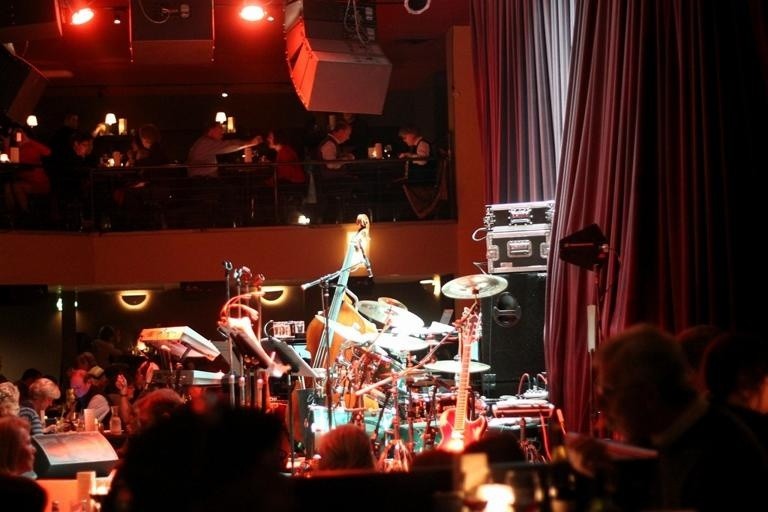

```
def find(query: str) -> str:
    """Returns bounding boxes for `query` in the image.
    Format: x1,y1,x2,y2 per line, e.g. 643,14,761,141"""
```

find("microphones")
355,239,378,279
354,342,392,369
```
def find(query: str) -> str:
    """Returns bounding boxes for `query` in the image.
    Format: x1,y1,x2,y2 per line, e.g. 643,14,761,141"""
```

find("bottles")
107,405,123,433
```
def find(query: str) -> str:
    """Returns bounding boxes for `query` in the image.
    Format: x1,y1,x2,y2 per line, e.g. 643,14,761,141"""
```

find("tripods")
336,373,437,474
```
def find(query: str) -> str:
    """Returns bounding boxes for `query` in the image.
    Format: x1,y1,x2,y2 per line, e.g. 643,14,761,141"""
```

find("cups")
76,470,96,502
83,408,97,432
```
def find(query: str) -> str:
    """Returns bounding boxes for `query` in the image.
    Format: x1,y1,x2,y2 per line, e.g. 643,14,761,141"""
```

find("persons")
2,320,768,512
252,127,309,226
397,124,440,221
187,120,263,227
0,112,176,225
318,121,373,222
344,113,368,138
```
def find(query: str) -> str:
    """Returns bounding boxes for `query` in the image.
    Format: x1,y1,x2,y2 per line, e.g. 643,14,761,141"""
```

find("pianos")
491,402,554,418
151,370,223,386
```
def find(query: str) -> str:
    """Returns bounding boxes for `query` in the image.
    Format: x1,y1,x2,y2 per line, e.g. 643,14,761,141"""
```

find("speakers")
30,430,122,479
481,273,549,397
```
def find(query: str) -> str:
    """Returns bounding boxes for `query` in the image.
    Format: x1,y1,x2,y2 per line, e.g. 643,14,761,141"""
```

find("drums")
354,300,425,337
377,331,431,367
348,345,407,410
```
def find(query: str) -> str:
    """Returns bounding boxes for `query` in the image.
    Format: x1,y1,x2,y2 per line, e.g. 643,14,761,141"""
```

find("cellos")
284,212,380,446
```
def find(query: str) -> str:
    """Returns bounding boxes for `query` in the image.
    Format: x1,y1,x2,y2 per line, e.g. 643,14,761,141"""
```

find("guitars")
434,313,487,452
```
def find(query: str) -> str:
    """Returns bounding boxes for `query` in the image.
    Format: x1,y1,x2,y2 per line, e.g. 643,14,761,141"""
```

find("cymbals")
441,274,508,299
422,356,492,374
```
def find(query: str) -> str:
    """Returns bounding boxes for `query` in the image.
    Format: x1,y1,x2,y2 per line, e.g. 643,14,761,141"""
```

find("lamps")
120,290,149,309
256,286,287,305
